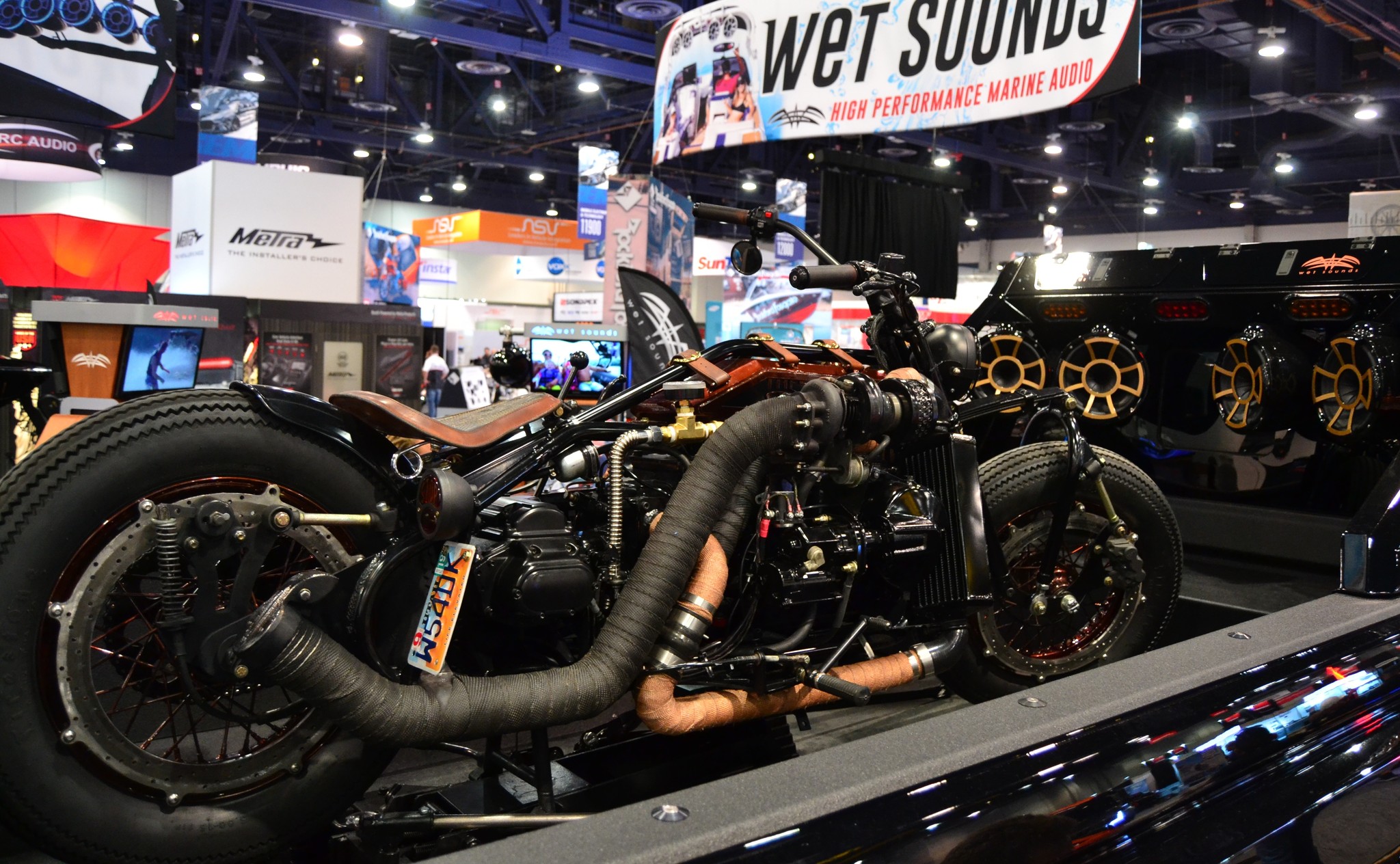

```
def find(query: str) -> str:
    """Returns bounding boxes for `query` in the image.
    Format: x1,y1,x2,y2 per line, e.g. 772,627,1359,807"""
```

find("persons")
530,341,619,393
421,346,451,417
143,341,170,391
648,58,769,164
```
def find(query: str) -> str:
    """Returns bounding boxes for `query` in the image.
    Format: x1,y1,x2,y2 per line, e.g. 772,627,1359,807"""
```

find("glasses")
544,353,552,356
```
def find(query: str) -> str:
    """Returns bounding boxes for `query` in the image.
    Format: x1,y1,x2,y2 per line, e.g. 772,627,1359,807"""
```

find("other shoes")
424,412,430,417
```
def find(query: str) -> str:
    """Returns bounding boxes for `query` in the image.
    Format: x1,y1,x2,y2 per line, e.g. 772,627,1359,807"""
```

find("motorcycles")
0,204,1185,864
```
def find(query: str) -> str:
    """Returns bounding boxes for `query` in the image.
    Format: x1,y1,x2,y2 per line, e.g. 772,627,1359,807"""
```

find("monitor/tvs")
527,335,627,400
112,322,205,396
